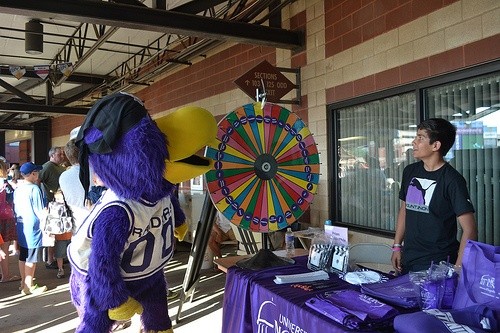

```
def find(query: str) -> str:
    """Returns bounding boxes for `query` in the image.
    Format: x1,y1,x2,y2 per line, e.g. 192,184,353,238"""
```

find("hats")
66,125,81,144
19,162,43,174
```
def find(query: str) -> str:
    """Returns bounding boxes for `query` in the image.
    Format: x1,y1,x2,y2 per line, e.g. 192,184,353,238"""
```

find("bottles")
285,228,295,257
323,220,332,232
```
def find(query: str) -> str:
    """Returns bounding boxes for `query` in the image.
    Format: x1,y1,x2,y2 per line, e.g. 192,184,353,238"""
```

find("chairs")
348,242,394,265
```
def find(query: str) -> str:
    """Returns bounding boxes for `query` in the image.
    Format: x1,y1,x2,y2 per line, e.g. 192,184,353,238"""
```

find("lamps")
25,19,76,55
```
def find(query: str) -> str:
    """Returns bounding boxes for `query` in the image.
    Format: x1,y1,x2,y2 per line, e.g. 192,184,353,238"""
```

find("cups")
413,268,459,310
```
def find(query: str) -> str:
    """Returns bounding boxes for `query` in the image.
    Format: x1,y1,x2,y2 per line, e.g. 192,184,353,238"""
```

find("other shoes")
45,260,64,270
19,283,47,296
56,271,65,279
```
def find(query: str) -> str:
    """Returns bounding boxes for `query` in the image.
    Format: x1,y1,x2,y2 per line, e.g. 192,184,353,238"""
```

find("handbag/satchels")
304,240,500,333
40,189,72,235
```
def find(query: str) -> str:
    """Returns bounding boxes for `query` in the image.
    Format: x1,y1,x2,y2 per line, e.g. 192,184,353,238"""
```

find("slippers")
0,271,22,283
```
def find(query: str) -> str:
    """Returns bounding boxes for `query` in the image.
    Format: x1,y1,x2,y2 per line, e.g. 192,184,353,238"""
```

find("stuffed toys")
65,91,218,333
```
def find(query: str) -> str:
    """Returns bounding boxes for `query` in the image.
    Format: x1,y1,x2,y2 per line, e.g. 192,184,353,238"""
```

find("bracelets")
391,244,402,252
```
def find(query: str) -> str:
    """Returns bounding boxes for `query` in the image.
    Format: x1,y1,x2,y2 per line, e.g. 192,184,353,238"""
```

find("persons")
391,118,476,274
0,126,107,297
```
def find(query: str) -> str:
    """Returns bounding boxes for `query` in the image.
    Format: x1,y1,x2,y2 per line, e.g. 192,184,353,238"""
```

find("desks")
213,249,397,333
293,228,354,250
